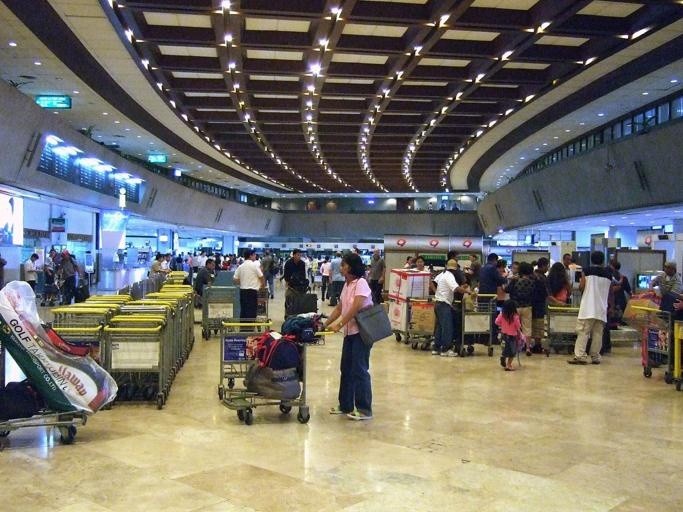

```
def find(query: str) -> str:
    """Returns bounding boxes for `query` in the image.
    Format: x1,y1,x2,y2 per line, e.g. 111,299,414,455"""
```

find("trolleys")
391,291,583,356
48,284,197,410
203,284,239,340
165,270,190,285
307,266,322,291
216,315,272,401
220,322,335,425
253,287,271,332
629,304,674,384
0,315,90,444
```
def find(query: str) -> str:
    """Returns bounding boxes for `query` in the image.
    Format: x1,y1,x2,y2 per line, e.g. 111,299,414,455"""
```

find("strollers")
36,264,66,307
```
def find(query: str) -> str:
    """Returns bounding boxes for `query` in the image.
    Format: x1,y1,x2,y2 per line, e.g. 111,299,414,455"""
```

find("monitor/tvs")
634,271,665,292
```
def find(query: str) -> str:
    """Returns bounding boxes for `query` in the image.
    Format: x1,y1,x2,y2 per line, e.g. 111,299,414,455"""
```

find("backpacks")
288,279,311,294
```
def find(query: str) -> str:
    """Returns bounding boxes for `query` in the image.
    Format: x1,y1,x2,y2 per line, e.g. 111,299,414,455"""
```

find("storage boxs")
622,295,658,329
406,301,436,336
385,298,408,335
387,267,433,303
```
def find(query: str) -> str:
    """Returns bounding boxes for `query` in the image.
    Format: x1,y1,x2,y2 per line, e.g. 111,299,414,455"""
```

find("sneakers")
566,355,587,365
500,355,506,367
40,301,71,307
439,349,459,357
432,350,441,355
505,364,516,372
591,357,602,365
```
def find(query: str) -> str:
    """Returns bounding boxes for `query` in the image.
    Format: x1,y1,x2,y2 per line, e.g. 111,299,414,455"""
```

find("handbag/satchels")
622,290,663,330
280,312,328,344
242,330,303,401
2,378,44,420
74,284,90,303
352,303,394,349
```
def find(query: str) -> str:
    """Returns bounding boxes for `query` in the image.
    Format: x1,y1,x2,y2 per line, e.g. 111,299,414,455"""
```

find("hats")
446,259,458,271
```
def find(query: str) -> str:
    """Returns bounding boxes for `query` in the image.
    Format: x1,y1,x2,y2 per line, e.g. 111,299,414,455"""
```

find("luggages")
285,294,318,314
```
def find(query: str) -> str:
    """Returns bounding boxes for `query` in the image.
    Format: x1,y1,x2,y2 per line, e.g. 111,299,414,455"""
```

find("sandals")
328,405,354,414
347,410,373,422
